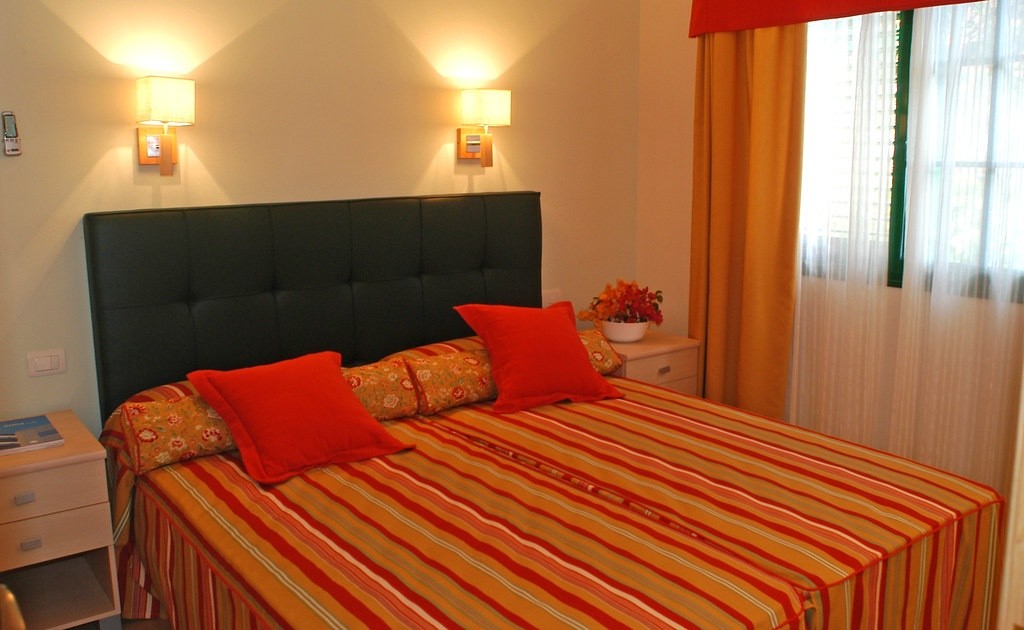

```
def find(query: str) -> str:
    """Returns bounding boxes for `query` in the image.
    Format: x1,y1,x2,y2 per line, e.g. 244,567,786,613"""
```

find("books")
0,415,64,455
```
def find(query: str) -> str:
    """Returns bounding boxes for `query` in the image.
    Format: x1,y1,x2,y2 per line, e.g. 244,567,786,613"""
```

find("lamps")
459,89,511,168
132,76,194,177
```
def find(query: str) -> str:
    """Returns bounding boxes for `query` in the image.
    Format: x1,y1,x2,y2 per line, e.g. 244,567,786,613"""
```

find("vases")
599,319,651,342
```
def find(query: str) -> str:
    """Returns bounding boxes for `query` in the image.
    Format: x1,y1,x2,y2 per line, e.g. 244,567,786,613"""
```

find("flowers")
579,278,666,328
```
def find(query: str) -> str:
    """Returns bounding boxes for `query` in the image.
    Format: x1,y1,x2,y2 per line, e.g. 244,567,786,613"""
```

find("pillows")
454,301,627,413
380,326,623,413
187,349,418,485
122,368,422,478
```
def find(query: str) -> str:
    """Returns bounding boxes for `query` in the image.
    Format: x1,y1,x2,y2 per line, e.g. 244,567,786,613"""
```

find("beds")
84,190,1024,630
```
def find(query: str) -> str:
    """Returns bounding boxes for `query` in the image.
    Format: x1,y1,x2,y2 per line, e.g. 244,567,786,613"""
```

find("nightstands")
0,410,127,630
609,329,702,400
